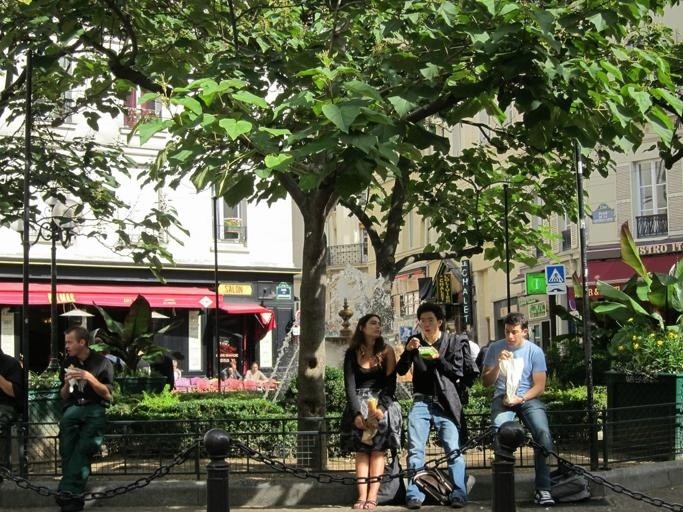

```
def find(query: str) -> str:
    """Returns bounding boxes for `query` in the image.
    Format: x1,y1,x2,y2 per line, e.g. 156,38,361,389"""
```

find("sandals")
353,500,378,511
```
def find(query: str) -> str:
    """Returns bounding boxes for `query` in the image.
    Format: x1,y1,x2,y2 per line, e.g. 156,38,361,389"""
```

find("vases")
606,372,683,461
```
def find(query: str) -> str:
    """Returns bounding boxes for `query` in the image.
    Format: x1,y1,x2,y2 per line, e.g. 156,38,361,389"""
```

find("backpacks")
550,465,592,504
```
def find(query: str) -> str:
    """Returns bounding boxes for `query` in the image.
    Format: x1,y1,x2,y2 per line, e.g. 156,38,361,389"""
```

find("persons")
218,362,243,382
54,325,114,511
459,328,481,380
172,358,181,381
338,312,398,511
393,300,473,509
244,362,268,381
0,343,23,488
478,311,559,507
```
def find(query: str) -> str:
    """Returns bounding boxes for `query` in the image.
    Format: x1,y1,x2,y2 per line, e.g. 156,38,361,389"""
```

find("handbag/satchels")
377,457,407,505
415,468,453,504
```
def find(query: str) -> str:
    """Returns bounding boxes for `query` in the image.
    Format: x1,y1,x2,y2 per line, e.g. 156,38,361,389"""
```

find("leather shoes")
55,496,84,512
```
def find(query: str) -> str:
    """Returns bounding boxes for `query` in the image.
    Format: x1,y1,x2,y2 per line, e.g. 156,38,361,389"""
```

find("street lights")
10,180,84,375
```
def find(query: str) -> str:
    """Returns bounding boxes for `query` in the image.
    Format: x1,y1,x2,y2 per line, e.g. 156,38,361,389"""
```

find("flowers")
611,317,683,374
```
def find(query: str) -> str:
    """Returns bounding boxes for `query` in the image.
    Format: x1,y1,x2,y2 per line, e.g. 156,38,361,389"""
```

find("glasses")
420,319,433,324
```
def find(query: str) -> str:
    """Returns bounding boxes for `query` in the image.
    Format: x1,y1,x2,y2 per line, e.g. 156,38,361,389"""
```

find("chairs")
175,376,277,392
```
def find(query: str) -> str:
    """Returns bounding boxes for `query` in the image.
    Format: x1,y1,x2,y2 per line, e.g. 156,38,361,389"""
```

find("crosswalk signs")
545,264,566,286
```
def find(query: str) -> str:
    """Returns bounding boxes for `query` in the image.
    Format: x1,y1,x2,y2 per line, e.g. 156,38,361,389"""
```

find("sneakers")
534,491,555,505
451,498,465,507
407,498,420,509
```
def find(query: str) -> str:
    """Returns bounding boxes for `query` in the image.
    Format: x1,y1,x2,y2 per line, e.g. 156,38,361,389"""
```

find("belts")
68,398,101,404
414,395,439,400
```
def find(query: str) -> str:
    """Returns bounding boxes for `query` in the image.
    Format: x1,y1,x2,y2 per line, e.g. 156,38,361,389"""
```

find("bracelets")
516,394,525,406
